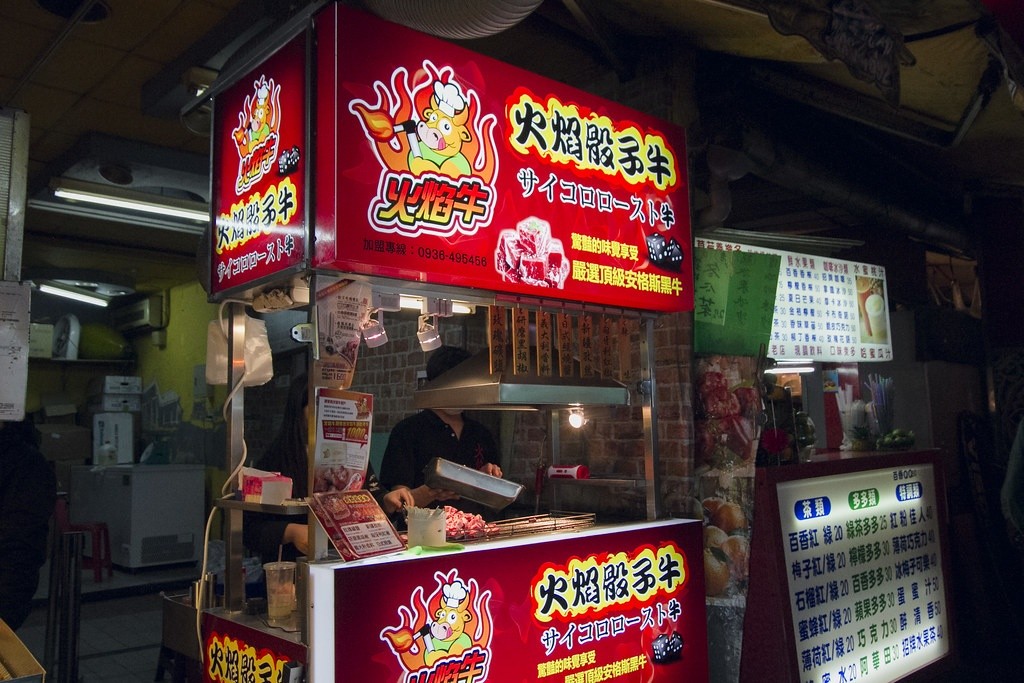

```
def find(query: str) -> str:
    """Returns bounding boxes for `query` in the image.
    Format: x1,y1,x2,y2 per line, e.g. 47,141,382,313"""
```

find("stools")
71,521,113,583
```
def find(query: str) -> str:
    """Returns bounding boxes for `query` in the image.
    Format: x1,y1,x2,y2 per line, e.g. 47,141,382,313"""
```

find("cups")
866,294,887,344
857,279,871,335
408,509,447,548
839,410,864,442
865,409,891,446
263,560,297,620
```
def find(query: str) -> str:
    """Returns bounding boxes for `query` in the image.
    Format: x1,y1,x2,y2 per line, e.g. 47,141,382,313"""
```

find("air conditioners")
113,295,162,336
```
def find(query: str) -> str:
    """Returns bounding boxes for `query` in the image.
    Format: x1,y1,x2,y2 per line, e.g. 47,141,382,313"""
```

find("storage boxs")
94,413,134,466
29,323,54,358
102,394,141,412
35,424,92,460
103,376,144,395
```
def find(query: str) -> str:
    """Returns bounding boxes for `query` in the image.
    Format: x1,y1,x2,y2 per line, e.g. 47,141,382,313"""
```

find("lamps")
54,187,209,222
38,284,109,308
362,309,388,348
417,315,442,352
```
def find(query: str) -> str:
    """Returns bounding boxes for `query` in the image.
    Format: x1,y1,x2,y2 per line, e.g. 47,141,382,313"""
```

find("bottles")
99,441,117,465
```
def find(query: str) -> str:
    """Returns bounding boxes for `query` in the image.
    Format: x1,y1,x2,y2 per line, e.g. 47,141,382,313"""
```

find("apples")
698,496,748,599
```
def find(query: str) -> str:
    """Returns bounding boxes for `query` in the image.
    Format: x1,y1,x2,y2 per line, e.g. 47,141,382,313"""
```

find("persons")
381,347,503,523
248,370,414,566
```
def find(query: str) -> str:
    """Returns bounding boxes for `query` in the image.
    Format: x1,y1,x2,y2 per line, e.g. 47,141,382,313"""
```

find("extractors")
412,307,631,414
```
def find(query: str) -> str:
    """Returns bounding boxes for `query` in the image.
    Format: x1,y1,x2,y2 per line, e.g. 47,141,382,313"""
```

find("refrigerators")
70,459,206,569
